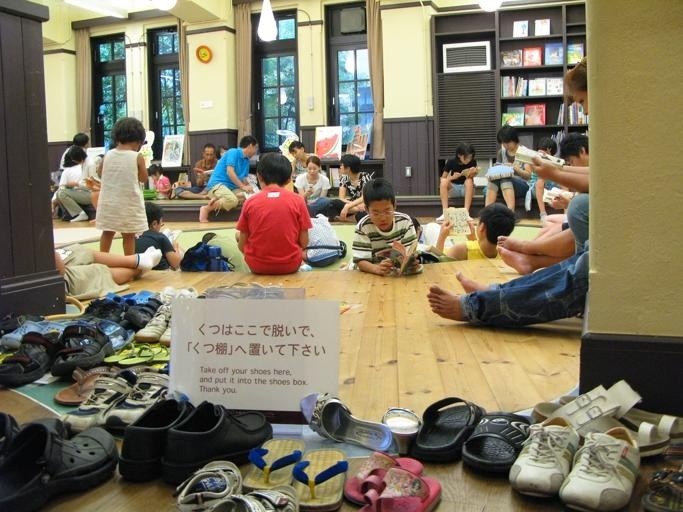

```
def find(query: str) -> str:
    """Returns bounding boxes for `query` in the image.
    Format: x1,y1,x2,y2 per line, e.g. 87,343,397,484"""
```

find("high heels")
382,406,421,456
300,392,400,454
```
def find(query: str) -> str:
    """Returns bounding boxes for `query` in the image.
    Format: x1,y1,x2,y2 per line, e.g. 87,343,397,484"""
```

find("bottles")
207,246,223,272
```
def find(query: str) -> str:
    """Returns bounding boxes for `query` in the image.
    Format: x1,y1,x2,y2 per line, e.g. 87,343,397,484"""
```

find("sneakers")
118,398,196,483
1,282,198,432
508,416,580,499
159,401,274,488
559,427,641,512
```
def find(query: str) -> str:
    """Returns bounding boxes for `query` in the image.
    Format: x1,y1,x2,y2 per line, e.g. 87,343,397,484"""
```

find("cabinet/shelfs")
428,2,587,195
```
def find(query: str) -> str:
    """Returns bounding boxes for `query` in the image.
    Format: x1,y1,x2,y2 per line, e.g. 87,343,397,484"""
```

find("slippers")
291,447,347,512
531,385,670,458
0,412,72,465
0,417,118,512
242,438,306,496
559,379,683,445
462,411,533,473
344,451,424,506
414,396,488,462
359,467,442,512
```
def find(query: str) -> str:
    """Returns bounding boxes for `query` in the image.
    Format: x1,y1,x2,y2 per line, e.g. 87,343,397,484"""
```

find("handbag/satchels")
180,243,231,273
340,240,347,257
487,165,514,180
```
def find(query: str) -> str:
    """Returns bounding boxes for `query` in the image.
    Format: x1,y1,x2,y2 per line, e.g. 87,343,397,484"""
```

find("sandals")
200,484,298,512
641,445,683,512
173,461,242,512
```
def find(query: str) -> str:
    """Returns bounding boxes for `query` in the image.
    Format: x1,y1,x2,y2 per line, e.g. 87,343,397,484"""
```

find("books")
499,18,585,68
